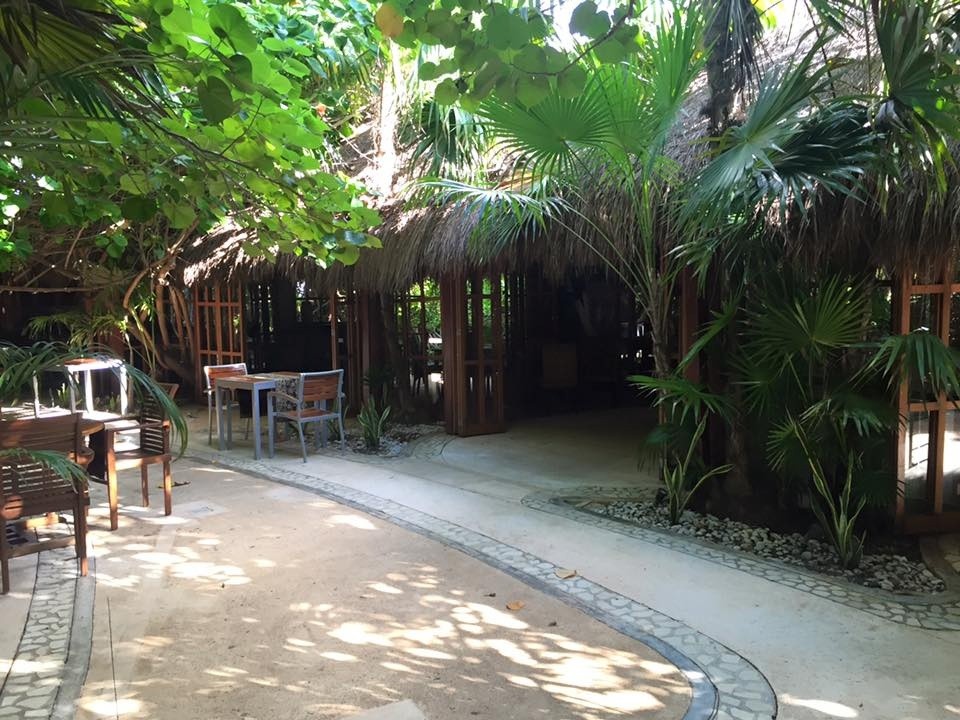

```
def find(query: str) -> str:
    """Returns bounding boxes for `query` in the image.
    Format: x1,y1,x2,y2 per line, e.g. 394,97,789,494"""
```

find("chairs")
0,410,92,596
94,380,181,531
203,361,249,443
266,367,354,464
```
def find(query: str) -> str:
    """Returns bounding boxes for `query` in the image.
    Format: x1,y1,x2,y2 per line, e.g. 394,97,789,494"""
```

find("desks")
81,411,103,439
213,370,311,460
32,354,131,415
